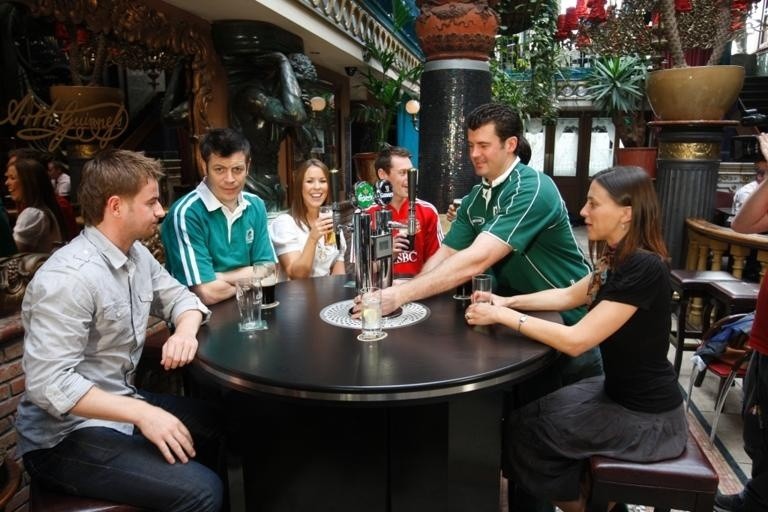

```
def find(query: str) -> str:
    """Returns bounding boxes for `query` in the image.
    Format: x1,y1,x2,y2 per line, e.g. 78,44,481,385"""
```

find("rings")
465,312,471,319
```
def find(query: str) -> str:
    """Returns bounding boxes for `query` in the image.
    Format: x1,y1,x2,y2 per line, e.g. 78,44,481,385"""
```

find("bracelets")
306,235,318,242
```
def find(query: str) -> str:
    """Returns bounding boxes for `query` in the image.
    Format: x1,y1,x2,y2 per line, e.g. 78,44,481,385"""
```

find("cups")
236,277,263,331
252,261,277,308
455,280,471,300
319,206,336,245
359,288,385,339
471,274,493,306
399,228,409,251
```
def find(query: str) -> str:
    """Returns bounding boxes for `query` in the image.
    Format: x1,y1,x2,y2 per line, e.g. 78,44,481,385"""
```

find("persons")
267,157,347,284
160,127,278,307
229,49,319,214
351,102,603,409
463,165,687,511
16,147,229,512
350,146,443,286
713,130,767,511
725,152,767,224
0,150,73,257
445,136,531,223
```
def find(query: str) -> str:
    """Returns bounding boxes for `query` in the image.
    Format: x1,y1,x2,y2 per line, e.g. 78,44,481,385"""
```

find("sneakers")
713,479,768,512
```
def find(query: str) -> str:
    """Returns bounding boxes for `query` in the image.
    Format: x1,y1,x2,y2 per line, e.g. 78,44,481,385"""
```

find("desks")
1,451,24,510
192,275,567,511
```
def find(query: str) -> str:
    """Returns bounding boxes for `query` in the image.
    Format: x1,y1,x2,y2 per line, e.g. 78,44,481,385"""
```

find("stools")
668,268,739,380
26,470,143,511
144,324,187,396
587,429,718,510
694,280,761,410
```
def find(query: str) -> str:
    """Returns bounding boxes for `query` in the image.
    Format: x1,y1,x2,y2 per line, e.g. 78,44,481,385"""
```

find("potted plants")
588,53,658,179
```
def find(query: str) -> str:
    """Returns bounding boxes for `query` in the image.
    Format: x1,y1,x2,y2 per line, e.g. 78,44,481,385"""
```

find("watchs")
517,313,528,335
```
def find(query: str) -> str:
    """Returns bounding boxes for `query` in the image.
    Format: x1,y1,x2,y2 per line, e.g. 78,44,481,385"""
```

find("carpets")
564,382,751,512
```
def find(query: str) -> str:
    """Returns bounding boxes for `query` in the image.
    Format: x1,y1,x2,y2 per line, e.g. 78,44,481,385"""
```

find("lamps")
306,96,326,114
407,99,419,133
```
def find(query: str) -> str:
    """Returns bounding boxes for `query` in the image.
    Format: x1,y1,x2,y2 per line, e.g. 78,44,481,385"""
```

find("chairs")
685,312,757,443
